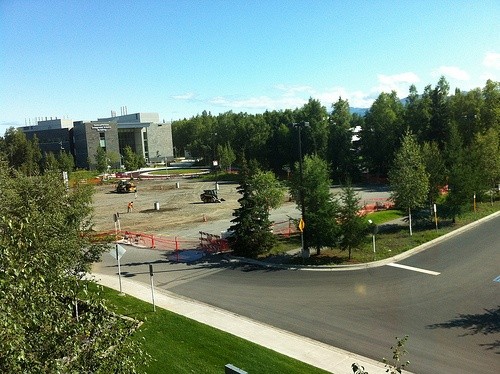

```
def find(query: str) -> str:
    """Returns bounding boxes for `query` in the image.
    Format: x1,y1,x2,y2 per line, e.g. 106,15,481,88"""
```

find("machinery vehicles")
200,188,226,204
116,180,137,194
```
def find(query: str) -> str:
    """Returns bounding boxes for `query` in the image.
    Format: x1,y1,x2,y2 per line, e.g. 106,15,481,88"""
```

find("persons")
127,201,133,213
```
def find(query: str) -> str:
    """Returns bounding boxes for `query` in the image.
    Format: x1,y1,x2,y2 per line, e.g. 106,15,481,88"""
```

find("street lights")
211,160,220,192
292,121,309,267
